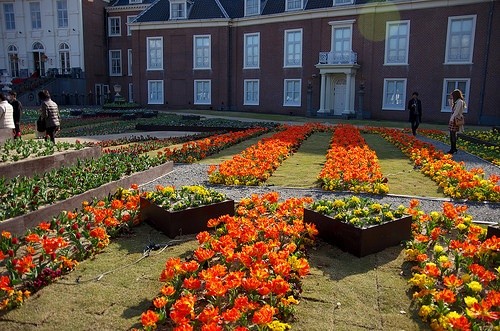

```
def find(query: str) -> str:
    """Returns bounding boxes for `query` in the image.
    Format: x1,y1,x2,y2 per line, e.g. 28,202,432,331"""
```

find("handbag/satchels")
454,117,464,125
37,115,47,132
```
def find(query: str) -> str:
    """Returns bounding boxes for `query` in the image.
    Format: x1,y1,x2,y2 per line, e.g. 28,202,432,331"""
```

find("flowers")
146,184,227,211
309,195,410,228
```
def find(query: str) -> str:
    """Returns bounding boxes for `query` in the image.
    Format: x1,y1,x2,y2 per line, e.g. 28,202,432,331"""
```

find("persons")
0,91,22,143
408,92,422,137
447,89,466,154
38,90,61,144
35,63,41,79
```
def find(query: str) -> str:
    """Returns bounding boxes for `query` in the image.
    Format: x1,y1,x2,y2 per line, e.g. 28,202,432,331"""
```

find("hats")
8,91,16,95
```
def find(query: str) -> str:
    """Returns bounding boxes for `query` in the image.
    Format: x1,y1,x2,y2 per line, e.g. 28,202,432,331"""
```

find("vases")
140,194,234,239
303,206,413,258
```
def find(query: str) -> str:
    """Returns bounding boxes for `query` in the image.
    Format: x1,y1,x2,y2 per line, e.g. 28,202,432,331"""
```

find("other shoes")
447,150,454,154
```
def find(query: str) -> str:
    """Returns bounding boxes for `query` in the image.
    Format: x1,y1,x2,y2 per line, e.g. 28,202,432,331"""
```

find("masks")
6,96,11,99
